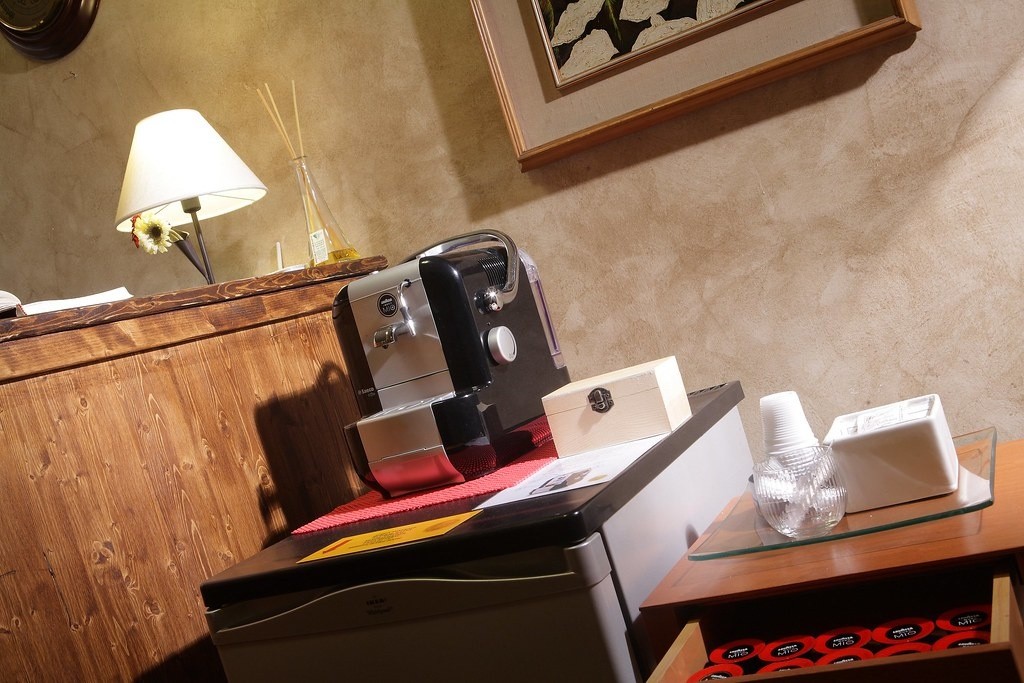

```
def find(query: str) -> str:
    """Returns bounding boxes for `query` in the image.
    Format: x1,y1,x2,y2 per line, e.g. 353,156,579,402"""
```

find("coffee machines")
332,228,569,500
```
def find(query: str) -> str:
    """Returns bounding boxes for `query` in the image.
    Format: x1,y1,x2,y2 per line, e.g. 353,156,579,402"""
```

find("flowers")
130,211,210,285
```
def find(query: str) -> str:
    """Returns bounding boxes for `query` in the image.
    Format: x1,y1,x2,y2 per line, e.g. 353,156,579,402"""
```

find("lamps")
114,106,267,284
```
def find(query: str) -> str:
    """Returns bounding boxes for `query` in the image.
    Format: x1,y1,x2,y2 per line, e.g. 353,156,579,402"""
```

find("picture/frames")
467,0,924,173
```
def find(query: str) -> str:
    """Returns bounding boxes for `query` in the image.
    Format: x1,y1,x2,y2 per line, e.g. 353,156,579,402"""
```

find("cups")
751,390,847,541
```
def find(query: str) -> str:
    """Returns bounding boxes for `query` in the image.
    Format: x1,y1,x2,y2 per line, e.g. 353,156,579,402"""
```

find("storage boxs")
541,354,692,458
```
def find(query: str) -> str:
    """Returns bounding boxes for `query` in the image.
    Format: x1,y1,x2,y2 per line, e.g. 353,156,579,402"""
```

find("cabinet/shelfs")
640,439,1024,683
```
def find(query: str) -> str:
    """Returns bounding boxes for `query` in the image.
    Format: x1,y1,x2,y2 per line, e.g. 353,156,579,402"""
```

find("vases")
290,155,359,267
